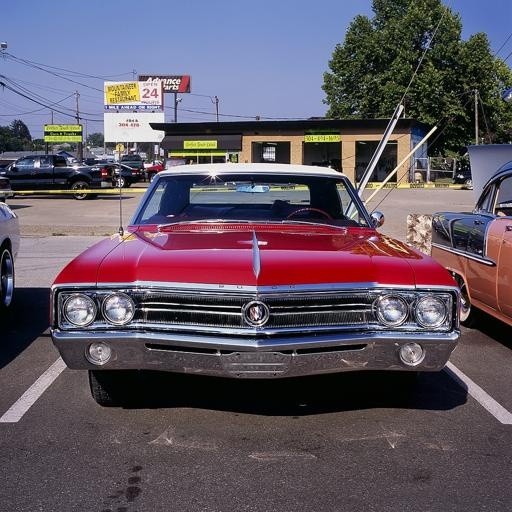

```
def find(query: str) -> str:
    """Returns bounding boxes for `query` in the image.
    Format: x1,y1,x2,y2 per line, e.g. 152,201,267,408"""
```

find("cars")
0,155,164,200
452,171,473,189
431,144,512,328
49,163,461,407
0,202,19,310
